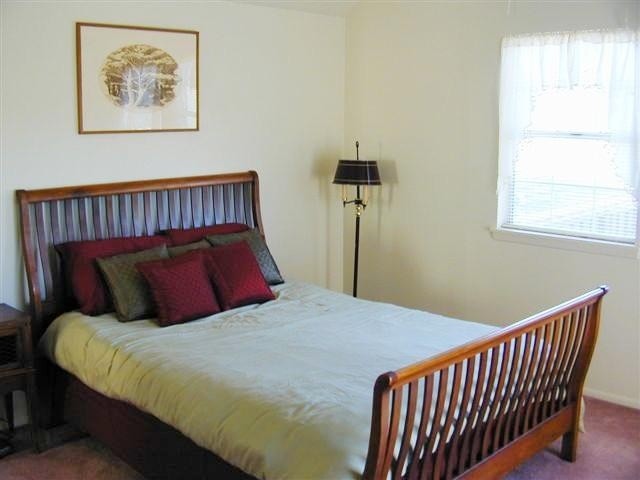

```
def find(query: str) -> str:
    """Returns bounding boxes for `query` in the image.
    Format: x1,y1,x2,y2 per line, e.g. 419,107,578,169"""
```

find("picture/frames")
76,22,200,133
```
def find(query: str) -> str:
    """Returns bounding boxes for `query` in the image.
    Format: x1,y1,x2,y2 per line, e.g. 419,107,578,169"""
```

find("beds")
14,171,611,479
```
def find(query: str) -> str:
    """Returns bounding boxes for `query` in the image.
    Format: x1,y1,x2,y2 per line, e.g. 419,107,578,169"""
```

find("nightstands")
0,304,41,461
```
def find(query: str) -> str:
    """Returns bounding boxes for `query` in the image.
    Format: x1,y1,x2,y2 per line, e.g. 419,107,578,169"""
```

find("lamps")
332,141,381,298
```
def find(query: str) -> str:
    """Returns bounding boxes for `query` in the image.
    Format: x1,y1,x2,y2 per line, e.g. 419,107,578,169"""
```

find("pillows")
94,241,173,322
168,237,212,259
204,227,285,286
138,252,222,327
162,221,245,249
52,235,173,318
201,242,278,312
